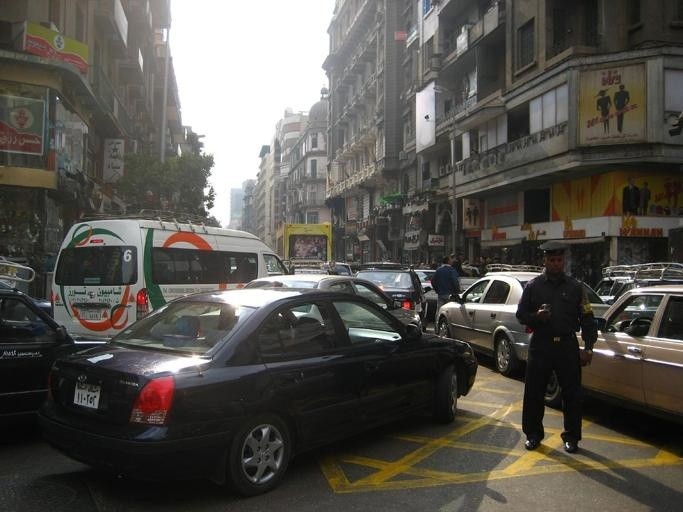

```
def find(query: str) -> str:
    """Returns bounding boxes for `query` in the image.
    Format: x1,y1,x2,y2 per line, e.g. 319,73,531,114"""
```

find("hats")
540,242,568,256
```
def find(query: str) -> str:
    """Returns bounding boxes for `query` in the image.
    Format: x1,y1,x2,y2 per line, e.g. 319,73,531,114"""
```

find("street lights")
427,81,458,255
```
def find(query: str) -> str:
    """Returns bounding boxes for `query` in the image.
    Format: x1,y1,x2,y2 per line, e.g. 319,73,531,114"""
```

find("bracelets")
585,347,595,355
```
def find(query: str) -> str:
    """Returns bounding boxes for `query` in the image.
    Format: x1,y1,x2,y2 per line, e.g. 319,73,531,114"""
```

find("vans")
45,207,294,345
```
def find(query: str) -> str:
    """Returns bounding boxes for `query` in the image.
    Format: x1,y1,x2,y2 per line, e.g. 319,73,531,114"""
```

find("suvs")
594,262,682,306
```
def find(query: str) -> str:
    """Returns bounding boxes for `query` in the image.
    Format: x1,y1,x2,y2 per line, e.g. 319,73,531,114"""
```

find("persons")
431,255,461,322
515,241,598,453
429,253,500,278
473,205,479,225
639,181,651,216
623,176,639,215
465,207,472,223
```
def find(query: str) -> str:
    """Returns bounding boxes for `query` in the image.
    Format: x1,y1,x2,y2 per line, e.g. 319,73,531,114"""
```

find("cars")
0,285,76,430
355,270,429,325
33,284,481,500
432,264,616,378
0,254,53,323
277,258,439,305
543,285,683,422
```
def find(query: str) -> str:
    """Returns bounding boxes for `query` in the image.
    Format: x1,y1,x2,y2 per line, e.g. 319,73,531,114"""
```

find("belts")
553,336,560,344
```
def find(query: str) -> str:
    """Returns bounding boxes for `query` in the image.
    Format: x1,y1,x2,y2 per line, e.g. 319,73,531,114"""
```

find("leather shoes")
524,439,539,450
564,441,577,452
